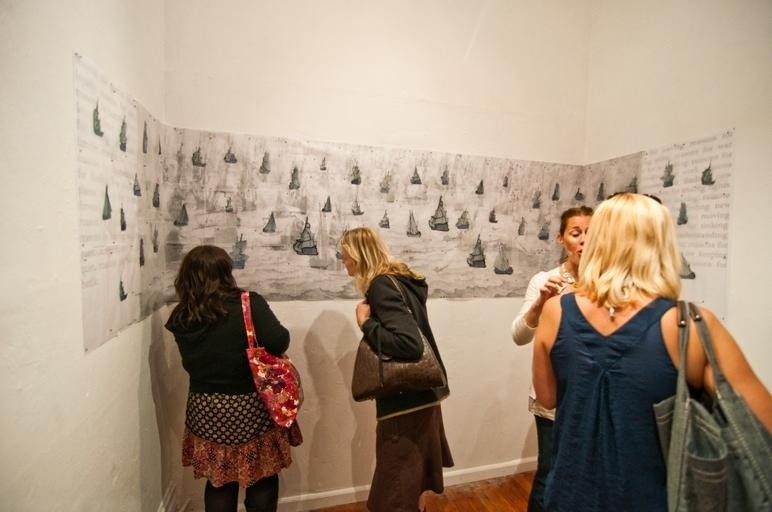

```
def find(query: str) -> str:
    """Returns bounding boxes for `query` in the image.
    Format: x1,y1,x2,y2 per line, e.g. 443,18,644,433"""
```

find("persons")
164,244,290,510
530,192,772,512
337,226,454,512
511,204,594,506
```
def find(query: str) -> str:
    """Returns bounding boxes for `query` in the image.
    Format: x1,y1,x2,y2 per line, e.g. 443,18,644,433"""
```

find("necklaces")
560,261,576,285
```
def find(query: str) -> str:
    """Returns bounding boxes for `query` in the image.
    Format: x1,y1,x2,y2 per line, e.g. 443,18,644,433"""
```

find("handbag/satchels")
652,385,772,512
352,331,444,402
245,346,304,430
